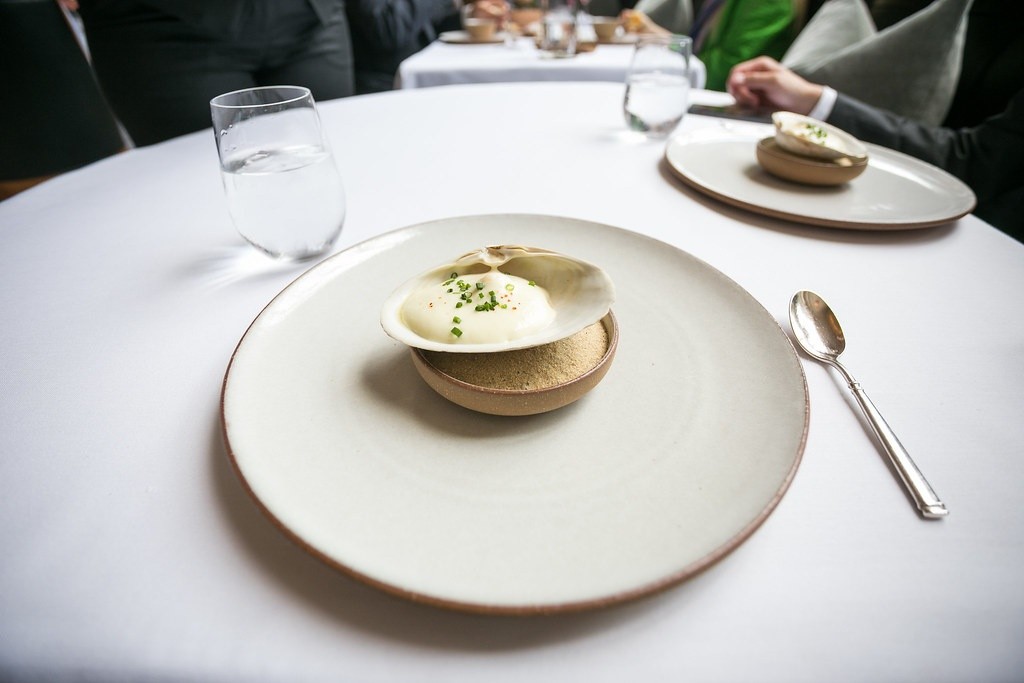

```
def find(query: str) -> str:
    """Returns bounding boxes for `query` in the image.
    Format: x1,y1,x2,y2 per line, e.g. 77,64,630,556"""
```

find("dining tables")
0,81,1023,683
392,34,707,91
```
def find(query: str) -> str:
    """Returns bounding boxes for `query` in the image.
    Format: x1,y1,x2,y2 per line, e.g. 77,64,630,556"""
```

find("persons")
1,0,518,206
614,0,808,93
724,50,1024,244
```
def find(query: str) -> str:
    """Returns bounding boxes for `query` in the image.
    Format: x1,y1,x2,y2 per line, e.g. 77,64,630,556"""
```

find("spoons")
790,290,950,517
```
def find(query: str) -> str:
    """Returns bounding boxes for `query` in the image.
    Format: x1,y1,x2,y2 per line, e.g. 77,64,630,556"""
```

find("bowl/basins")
411,304,617,415
759,136,868,184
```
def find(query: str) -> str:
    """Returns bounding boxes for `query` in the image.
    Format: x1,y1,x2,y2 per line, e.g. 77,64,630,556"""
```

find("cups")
542,0,575,54
625,37,691,133
466,18,495,38
592,22,617,42
210,87,344,259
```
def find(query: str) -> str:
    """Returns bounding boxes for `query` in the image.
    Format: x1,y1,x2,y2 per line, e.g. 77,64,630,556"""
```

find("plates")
441,30,506,43
665,123,976,228
220,214,808,613
